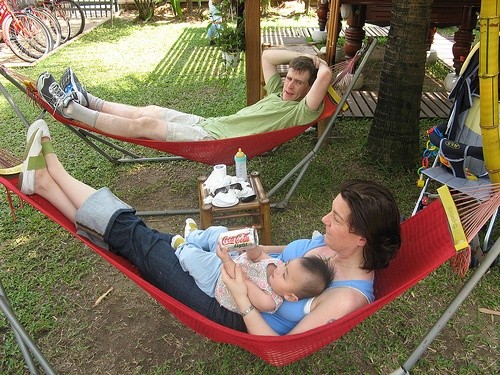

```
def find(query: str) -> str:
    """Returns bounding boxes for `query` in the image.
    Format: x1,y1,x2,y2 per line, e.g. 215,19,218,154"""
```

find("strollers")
410,41,500,255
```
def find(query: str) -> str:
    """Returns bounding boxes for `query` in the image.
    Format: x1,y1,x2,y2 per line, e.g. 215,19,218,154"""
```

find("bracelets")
241,307,254,317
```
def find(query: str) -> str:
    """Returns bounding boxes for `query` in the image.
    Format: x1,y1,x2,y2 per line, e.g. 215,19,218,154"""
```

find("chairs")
411,102,500,251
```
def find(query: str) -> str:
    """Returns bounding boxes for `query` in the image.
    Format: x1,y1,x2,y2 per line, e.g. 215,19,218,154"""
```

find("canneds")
218,228,259,252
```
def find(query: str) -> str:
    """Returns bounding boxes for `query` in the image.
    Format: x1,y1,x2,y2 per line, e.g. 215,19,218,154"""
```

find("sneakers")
60,67,89,108
36,71,79,120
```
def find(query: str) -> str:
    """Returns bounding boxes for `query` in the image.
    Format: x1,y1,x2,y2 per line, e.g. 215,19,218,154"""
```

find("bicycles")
0,0,86,65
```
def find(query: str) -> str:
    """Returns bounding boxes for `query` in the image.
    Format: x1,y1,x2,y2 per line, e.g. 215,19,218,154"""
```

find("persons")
38,50,331,164
18,118,402,365
171,218,337,315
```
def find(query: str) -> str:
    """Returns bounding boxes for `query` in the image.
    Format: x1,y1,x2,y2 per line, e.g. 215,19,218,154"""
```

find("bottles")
234,148,247,181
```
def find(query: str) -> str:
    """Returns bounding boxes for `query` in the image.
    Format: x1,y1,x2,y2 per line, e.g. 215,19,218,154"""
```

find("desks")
198,171,272,245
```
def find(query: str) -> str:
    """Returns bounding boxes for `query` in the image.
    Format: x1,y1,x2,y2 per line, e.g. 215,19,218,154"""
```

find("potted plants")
214,24,241,67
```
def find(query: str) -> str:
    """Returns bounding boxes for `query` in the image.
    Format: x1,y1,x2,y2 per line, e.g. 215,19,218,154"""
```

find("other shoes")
27,119,54,156
184,218,198,239
18,128,47,195
171,235,186,250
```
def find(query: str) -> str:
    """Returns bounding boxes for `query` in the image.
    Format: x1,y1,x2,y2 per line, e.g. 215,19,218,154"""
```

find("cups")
213,164,226,181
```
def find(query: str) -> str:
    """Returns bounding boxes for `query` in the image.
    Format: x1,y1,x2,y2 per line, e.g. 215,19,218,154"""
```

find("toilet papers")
202,162,228,196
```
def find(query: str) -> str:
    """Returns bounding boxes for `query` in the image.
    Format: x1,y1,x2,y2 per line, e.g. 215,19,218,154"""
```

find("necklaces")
322,256,331,261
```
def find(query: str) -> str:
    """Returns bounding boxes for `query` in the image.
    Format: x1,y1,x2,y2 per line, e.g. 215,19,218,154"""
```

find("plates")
212,196,239,207
